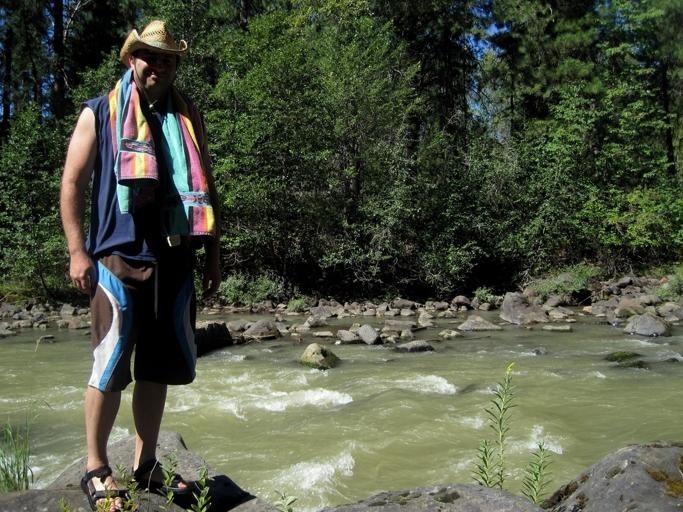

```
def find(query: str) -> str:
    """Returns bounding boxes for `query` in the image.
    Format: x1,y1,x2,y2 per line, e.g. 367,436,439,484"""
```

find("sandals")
131,459,191,496
80,464,131,512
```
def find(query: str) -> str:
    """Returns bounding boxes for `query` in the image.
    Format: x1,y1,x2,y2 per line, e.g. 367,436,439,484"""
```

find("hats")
120,20,189,70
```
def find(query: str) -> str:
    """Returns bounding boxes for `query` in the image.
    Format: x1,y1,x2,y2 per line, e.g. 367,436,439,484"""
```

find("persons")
57,17,223,511
